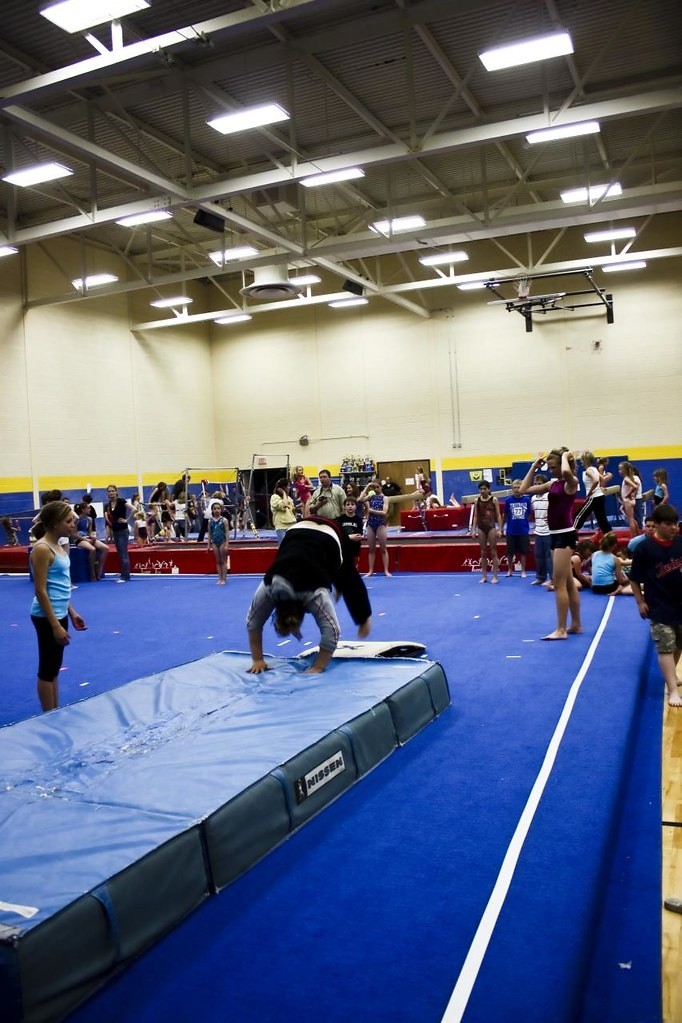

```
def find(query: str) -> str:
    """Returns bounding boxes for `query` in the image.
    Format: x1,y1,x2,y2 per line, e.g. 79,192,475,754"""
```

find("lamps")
113,131,174,227
477,0,574,73
33,0,152,34
204,50,293,135
525,121,601,145
343,274,369,296
0,245,19,257
601,259,647,273
582,220,636,243
1,98,74,188
560,145,622,204
208,198,259,262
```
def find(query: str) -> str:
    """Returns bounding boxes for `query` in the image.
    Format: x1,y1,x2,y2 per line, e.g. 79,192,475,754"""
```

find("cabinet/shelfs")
339,471,376,488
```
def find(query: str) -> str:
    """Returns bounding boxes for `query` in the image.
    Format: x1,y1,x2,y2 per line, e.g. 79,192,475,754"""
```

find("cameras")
319,496,327,501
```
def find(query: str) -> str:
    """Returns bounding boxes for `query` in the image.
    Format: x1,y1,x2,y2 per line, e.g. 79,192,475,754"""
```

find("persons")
519,446,584,640
270,465,461,578
41,485,137,583
29,500,89,711
627,504,682,707
131,475,252,548
466,451,670,595
206,503,229,586
245,516,372,674
3,517,21,547
